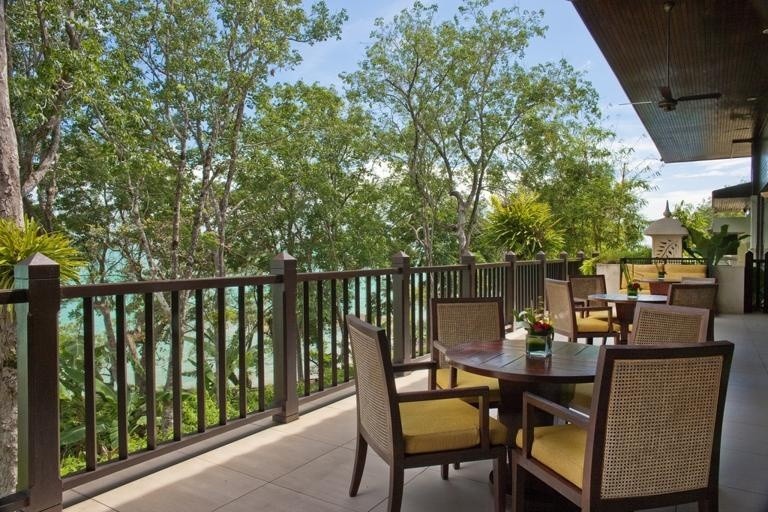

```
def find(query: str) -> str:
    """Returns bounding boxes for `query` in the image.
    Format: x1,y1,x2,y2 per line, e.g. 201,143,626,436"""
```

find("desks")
443,338,600,497
587,293,667,344
631,277,681,295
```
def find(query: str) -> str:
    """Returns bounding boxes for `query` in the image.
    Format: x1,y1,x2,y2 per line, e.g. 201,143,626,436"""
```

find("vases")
522,332,554,361
626,288,639,298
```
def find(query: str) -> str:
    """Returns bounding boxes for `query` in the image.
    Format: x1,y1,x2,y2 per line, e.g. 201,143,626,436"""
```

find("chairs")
348,314,512,511
567,274,617,345
665,282,720,341
427,295,510,471
681,275,716,286
544,277,621,345
569,302,715,418
512,339,735,511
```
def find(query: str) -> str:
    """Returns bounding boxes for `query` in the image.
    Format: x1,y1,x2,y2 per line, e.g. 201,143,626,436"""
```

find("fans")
619,1,723,113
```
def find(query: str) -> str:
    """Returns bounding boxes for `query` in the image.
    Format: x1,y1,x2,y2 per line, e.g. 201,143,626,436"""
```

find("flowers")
514,295,558,344
626,275,644,292
